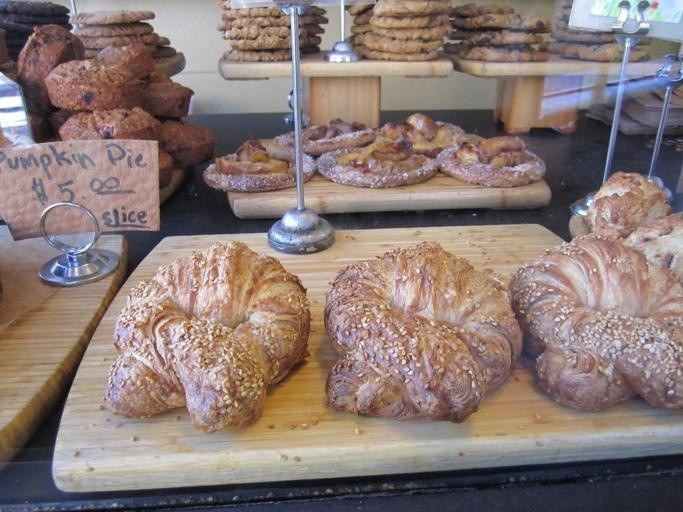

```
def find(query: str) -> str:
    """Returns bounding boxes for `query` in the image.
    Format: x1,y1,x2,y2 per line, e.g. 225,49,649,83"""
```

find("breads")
443,3,553,62
348,1,454,61
1,0,216,190
324,240,524,425
544,2,652,63
508,172,683,425
105,239,311,431
202,112,546,188
216,2,327,62
